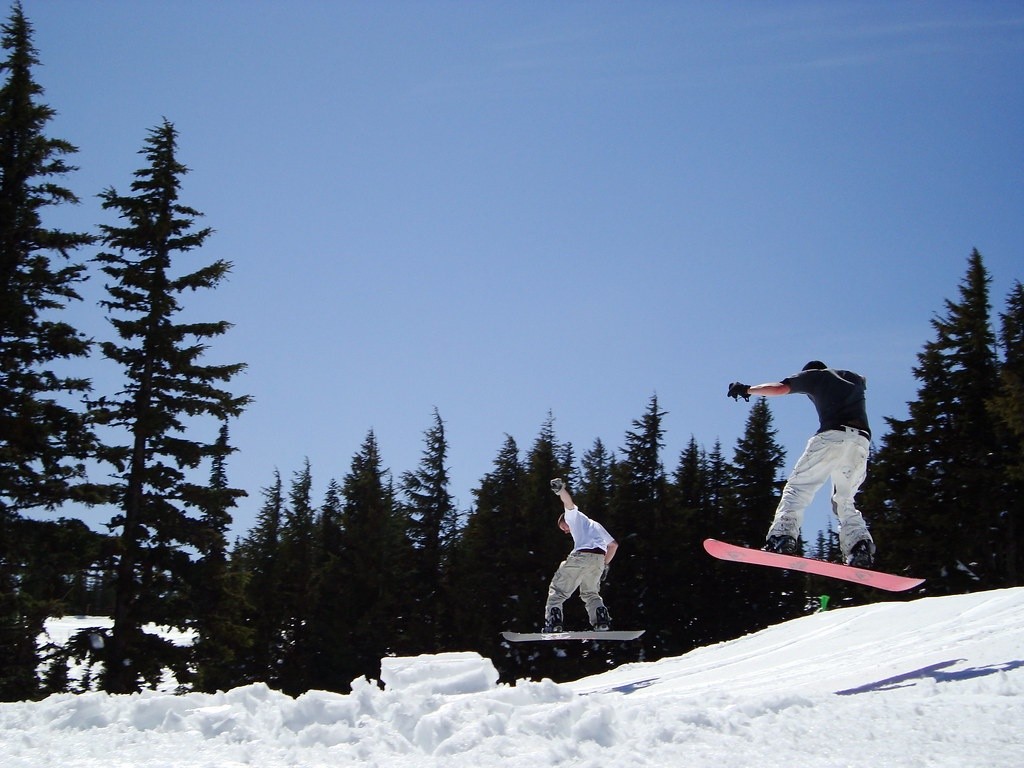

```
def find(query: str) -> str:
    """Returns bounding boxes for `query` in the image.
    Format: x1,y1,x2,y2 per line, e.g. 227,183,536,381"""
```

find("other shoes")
849,539,872,569
761,534,797,554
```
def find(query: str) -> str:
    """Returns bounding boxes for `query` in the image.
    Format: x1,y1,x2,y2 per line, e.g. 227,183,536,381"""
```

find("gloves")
550,478,564,496
601,564,610,581
727,382,752,402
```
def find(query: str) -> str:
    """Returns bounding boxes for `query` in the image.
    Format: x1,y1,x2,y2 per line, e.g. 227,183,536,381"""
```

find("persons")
541,477,619,633
726,360,876,569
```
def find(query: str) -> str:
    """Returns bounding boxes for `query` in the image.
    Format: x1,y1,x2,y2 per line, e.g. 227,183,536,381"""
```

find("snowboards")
702,537,927,593
501,627,648,643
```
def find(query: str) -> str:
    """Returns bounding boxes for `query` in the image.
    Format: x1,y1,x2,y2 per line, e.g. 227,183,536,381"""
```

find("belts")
818,425,871,440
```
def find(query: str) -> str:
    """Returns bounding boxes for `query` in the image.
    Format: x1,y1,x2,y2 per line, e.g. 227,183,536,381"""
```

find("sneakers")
592,606,609,631
542,607,563,633
